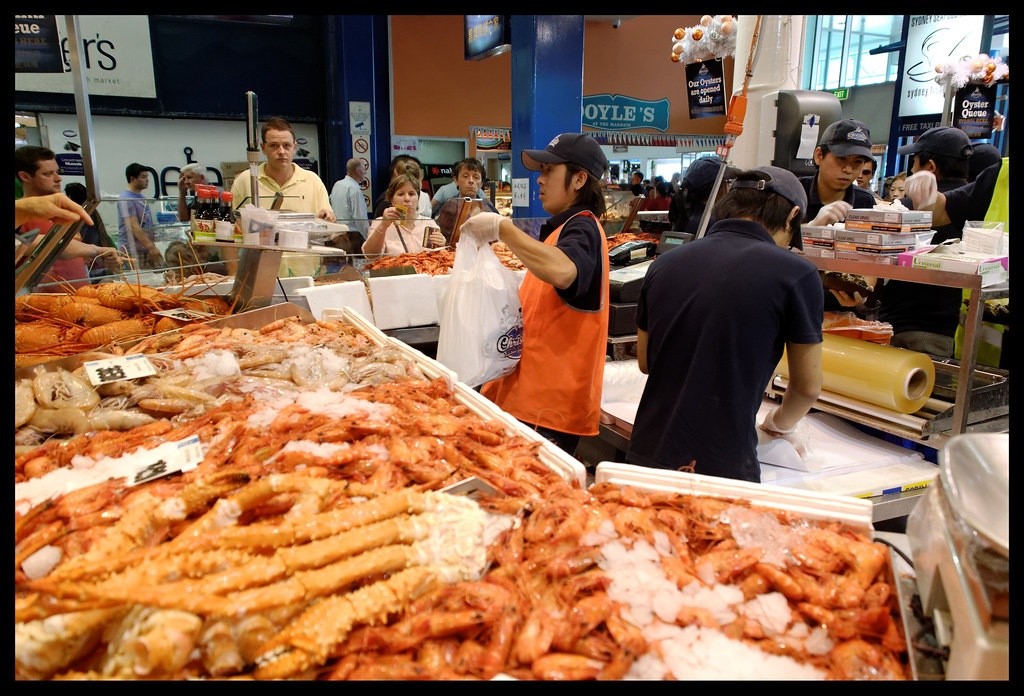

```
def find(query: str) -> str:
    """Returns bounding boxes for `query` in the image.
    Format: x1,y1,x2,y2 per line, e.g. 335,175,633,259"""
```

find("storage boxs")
798,208,937,265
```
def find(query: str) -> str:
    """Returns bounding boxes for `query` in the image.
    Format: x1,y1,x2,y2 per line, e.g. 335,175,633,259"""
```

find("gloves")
460,212,512,248
806,199,853,227
904,172,938,210
761,407,797,439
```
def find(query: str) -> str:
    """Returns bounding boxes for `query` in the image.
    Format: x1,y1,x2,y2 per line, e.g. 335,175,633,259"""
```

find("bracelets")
376,229,383,233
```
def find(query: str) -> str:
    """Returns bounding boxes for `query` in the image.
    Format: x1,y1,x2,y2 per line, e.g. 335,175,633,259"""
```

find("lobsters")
15,239,240,368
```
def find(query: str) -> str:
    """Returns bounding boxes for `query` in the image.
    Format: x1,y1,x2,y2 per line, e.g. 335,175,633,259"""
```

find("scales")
906,433,1010,681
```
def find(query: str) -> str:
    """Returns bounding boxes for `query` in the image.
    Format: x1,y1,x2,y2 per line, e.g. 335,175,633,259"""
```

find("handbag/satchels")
436,234,522,389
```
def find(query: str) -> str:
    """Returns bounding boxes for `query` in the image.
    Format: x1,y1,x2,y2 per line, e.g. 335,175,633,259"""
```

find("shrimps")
492,241,526,271
326,479,907,680
365,250,455,277
15,315,562,558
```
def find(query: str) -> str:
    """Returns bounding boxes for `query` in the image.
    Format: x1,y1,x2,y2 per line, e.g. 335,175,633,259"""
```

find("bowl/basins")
239,207,279,245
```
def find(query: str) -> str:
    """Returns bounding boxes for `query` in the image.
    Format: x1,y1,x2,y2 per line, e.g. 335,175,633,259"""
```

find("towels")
365,274,439,330
432,270,528,327
294,279,374,325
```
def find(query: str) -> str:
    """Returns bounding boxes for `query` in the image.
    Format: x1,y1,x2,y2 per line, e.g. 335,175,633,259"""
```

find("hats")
870,158,877,177
729,165,805,251
821,117,876,162
522,133,609,181
683,155,751,193
899,125,971,168
969,142,1002,179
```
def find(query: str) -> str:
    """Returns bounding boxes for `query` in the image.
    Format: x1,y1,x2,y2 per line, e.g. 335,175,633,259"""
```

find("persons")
231,117,336,276
177,163,211,222
627,166,824,483
374,154,432,217
361,174,446,261
460,133,610,459
14,146,121,293
618,171,680,260
795,119,1009,368
118,162,164,269
330,158,370,262
431,157,511,219
667,156,744,234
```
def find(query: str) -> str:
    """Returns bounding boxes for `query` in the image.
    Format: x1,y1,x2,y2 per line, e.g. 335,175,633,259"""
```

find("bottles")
190,184,236,243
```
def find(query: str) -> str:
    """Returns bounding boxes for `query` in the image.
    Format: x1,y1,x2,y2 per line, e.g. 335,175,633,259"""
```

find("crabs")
14,471,514,681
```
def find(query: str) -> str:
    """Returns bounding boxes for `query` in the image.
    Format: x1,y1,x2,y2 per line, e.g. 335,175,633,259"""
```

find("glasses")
862,169,873,176
